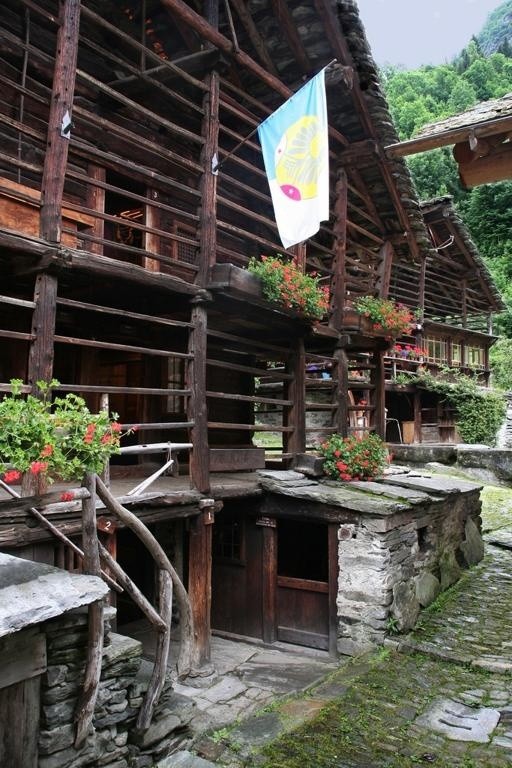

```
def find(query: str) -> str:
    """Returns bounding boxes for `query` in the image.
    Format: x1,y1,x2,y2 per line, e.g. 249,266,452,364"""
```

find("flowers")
351,296,424,341
316,430,396,483
382,344,433,361
244,252,333,320
0,373,143,502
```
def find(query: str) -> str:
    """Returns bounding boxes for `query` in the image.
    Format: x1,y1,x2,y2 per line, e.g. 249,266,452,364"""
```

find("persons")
350,368,367,438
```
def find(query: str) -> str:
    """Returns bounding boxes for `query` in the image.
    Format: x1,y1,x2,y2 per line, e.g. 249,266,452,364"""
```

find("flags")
254,68,329,246
